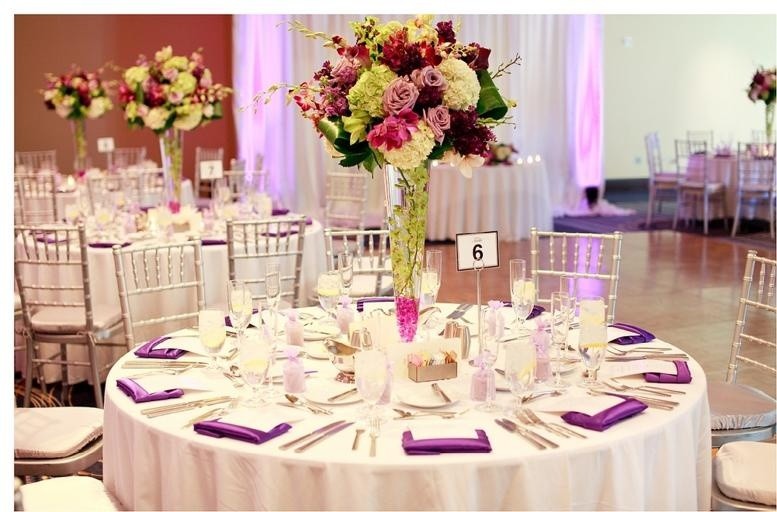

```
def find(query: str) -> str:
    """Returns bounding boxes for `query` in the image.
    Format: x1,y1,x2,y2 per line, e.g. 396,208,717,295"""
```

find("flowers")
746,64,776,105
240,14,526,173
116,44,235,135
37,61,114,119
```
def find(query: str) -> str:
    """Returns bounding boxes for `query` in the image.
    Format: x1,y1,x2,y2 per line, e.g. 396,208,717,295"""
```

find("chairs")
642,128,777,240
530,225,624,328
14,145,268,224
14,224,124,408
323,229,395,298
14,403,102,476
227,214,309,311
113,234,207,352
14,472,130,512
320,169,369,256
705,249,777,447
711,439,777,511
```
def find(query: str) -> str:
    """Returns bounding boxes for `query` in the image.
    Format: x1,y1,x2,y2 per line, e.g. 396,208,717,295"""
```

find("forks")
606,343,673,357
604,378,687,398
514,406,587,439
351,417,380,456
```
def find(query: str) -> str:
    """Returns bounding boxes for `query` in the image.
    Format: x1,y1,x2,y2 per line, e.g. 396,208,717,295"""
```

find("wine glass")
198,248,606,424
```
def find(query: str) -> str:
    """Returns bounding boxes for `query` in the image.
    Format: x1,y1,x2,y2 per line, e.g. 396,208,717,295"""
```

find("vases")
764,103,776,148
69,114,91,174
381,158,433,340
158,128,186,214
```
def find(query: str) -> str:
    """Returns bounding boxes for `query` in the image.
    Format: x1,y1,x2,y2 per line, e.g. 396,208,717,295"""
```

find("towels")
193,406,290,445
561,392,647,431
225,308,259,328
135,335,187,359
357,296,395,312
644,358,693,385
609,322,656,344
502,300,546,321
114,372,185,403
401,417,492,454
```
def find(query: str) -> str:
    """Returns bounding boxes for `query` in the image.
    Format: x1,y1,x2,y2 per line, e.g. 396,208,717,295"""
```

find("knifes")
494,418,559,451
606,354,689,362
121,359,206,369
280,420,355,454
141,394,233,418
